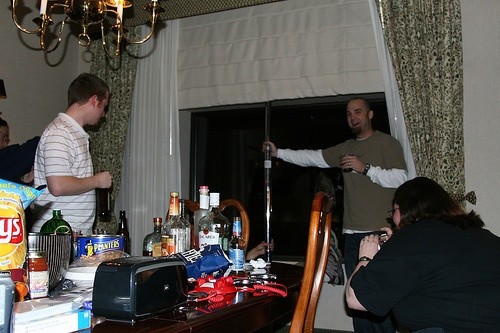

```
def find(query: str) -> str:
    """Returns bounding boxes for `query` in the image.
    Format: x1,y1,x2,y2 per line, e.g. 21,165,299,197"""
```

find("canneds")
75,234,125,259
27,251,50,300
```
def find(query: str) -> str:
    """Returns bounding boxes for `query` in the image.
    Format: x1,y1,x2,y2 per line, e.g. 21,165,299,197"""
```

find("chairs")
166,199,250,249
286,192,334,333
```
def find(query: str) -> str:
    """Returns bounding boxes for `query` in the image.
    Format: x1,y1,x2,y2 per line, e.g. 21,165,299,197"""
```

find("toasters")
92,255,187,322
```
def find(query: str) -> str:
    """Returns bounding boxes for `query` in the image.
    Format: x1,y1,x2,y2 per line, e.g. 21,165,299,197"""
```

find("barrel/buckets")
77,235,124,256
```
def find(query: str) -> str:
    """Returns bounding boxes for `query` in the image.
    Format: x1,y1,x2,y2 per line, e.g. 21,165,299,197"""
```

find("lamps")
13,0,162,53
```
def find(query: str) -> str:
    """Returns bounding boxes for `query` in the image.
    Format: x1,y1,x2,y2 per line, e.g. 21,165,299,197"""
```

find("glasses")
0,132,10,144
388,208,400,218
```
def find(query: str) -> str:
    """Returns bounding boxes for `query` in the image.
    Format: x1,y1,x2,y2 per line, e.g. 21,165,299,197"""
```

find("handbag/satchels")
165,242,233,281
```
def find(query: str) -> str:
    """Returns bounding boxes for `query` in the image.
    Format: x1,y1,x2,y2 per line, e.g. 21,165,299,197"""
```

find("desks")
0,254,307,333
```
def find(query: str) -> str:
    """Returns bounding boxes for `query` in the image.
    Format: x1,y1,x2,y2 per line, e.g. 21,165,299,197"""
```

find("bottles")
0,269,16,333
24,251,49,299
98,188,111,222
264,137,272,168
40,209,74,265
177,199,192,252
198,193,228,256
193,185,209,251
228,217,245,276
162,192,186,254
115,211,131,255
143,218,161,257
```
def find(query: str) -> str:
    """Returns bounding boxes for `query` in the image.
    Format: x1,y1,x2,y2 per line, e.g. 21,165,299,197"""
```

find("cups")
341,153,356,173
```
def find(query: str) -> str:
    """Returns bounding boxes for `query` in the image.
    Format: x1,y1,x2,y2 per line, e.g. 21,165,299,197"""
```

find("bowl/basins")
27,233,72,295
64,266,97,288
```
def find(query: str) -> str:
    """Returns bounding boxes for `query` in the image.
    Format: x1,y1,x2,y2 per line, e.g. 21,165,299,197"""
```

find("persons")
345,177,500,333
262,98,409,280
31,73,113,233
0,117,41,187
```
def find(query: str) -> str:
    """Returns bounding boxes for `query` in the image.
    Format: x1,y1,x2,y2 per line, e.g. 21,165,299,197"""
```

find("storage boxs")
0,273,94,333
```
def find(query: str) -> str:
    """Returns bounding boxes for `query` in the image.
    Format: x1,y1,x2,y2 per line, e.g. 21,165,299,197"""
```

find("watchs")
359,256,372,262
363,164,370,175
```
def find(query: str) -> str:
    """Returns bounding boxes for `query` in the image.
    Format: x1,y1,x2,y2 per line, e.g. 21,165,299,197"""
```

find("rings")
348,162,351,166
